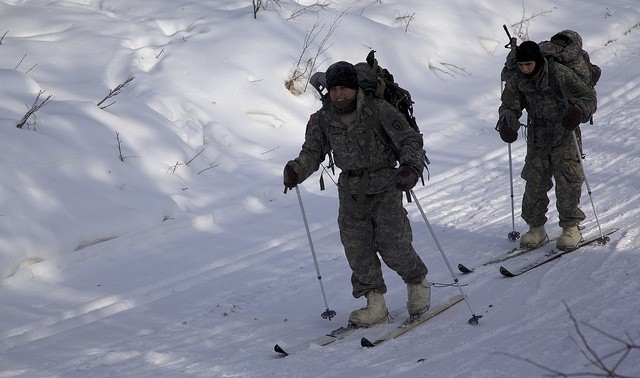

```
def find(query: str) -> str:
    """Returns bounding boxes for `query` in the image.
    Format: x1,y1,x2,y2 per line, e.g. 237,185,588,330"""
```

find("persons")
284,61,429,327
499,29,597,251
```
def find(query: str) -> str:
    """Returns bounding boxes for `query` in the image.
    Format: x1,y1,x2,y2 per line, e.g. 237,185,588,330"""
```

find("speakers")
556,226,584,250
349,292,388,325
520,226,546,247
406,279,430,315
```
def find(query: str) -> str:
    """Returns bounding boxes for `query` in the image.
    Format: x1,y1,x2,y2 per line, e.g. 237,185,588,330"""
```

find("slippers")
310,50,430,203
500,26,601,125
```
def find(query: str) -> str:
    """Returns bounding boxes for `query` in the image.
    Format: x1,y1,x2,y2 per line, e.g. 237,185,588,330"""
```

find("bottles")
326,62,358,91
515,41,544,77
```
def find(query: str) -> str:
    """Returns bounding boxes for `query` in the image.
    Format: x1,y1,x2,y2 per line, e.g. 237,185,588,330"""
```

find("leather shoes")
284,166,300,194
562,107,582,130
499,116,518,143
395,166,418,191
